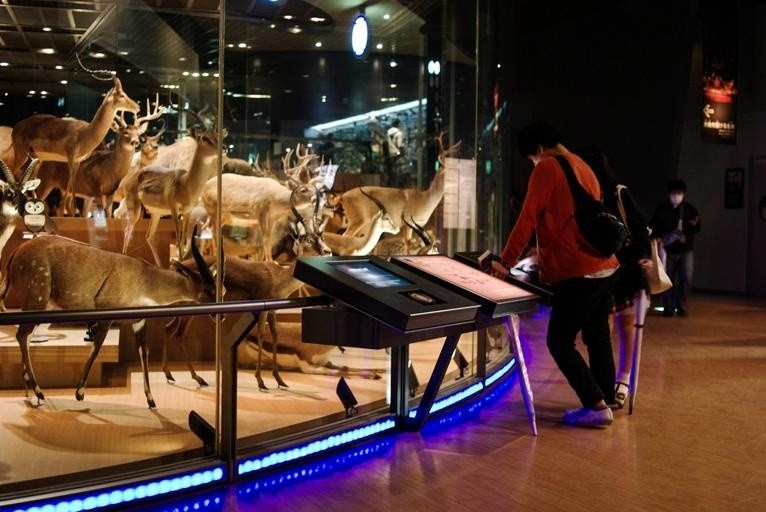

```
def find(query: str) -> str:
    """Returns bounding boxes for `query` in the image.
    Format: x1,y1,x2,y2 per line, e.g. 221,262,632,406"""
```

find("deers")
0,74,382,409
321,131,471,257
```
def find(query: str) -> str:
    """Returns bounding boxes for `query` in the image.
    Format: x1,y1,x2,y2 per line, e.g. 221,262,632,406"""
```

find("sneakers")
561,406,614,427
662,305,689,317
561,407,583,419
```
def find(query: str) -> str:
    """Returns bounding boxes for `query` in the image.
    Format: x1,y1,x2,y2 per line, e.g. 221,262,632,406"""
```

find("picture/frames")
725,168,744,208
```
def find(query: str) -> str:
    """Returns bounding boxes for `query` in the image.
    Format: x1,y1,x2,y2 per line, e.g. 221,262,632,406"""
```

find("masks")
670,194,683,205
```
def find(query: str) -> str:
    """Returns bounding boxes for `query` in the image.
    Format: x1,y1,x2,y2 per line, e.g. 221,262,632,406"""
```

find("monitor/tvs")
330,262,414,288
491,260,528,276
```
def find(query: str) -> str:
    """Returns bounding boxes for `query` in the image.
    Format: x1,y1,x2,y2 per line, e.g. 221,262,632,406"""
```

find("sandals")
612,379,630,411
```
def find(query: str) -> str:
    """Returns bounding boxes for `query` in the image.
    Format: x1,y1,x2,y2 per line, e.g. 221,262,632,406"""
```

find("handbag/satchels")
577,192,628,258
643,237,673,296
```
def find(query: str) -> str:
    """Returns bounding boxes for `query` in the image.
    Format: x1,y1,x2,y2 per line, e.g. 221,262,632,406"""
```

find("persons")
323,133,335,165
491,128,621,426
568,135,658,410
387,118,404,159
650,182,701,316
